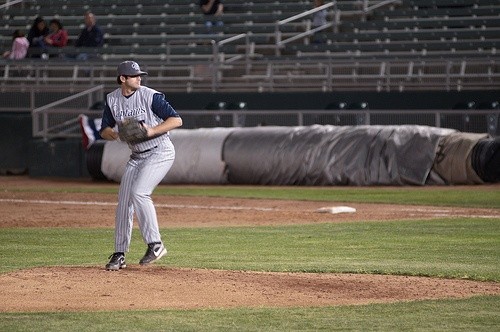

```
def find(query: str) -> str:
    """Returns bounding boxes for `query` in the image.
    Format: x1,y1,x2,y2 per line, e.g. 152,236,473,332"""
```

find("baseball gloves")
116,115,148,144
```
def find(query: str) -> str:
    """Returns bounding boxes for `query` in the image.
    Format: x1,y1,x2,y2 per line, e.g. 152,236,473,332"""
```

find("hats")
117,61,148,76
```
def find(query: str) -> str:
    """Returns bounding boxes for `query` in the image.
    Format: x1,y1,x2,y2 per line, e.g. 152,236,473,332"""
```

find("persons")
311,0,333,44
59,11,104,61
27,15,67,58
2,29,29,60
99,60,182,271
200,0,225,45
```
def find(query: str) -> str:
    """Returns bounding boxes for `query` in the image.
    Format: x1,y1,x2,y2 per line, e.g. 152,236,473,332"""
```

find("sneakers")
105,253,126,270
139,240,167,265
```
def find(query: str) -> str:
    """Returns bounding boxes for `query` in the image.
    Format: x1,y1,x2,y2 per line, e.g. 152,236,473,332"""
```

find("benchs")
0,0,500,91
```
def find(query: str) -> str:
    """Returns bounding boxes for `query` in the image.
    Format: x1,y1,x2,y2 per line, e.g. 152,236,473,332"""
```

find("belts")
135,145,157,154
